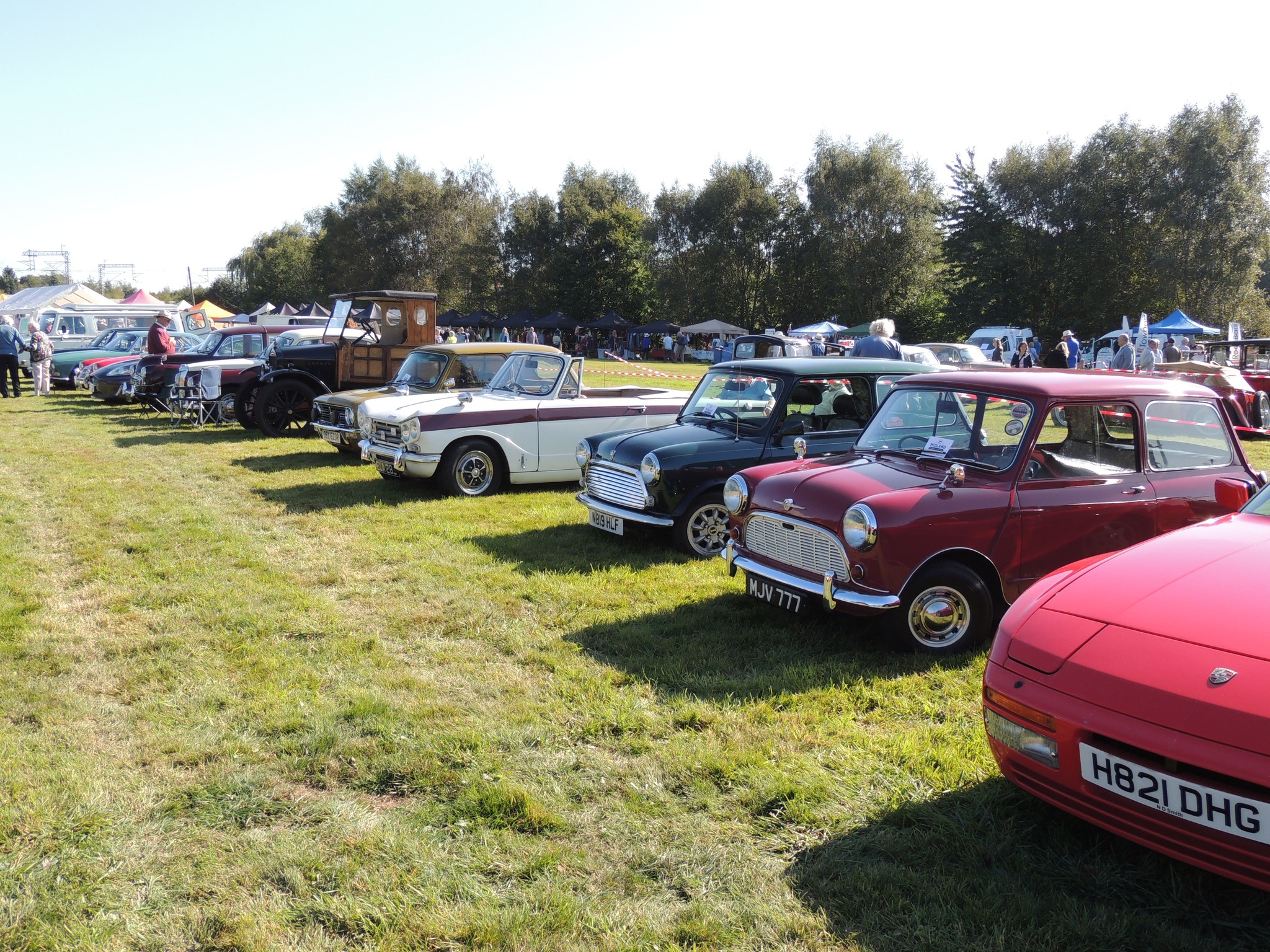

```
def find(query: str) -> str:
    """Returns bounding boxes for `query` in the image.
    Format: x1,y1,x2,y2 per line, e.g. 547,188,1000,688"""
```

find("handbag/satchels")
1035,358,1039,363
1076,360,1085,368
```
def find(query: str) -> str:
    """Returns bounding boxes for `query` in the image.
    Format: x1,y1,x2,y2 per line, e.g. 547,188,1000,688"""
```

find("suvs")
1051,361,1270,440
233,289,437,439
1202,338,1270,395
309,343,587,457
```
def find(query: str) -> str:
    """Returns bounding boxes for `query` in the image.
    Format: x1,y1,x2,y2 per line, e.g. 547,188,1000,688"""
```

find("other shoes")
45,393,49,396
35,394,40,396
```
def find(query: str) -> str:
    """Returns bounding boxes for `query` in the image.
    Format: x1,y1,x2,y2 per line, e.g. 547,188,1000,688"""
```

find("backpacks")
681,335,687,345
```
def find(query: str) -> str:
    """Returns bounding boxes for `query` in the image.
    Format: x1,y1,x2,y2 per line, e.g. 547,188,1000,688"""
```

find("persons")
0,315,25,398
716,375,776,416
18,320,54,398
991,330,1209,375
848,318,904,361
208,318,217,331
807,379,851,415
582,330,688,363
183,309,193,328
767,331,826,357
435,326,562,349
148,310,176,354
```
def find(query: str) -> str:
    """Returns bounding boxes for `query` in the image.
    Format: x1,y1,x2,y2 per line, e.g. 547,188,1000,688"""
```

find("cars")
128,324,382,424
913,343,1013,371
573,357,990,559
168,324,339,424
828,345,962,373
46,330,203,388
74,355,143,392
721,370,1270,660
87,336,278,405
982,477,1270,892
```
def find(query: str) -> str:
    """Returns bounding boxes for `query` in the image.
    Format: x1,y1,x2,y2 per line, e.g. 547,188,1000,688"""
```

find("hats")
1167,337,1176,343
775,331,785,336
1061,330,1074,340
153,310,175,322
1182,337,1189,342
644,333,650,337
812,333,825,343
117,318,124,323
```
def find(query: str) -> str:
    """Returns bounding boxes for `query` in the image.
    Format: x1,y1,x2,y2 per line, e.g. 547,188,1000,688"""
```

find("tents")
1,282,382,333
1132,308,1220,341
437,309,908,348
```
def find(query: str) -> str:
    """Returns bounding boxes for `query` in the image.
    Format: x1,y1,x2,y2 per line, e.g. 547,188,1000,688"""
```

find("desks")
565,348,713,362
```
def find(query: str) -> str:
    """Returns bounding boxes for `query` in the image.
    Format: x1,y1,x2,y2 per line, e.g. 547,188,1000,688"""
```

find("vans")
960,326,1032,364
14,303,211,378
1081,329,1185,370
731,332,813,360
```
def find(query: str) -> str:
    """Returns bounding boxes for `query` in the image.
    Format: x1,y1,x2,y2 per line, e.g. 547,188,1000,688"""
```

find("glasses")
164,316,170,322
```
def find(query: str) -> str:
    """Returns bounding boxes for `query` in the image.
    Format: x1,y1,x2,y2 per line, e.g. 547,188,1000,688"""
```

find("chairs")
778,384,822,434
170,365,226,430
129,368,189,420
826,394,867,432
401,328,408,344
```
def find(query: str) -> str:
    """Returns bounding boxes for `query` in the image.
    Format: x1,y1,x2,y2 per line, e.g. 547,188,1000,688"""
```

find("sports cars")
357,351,771,499
